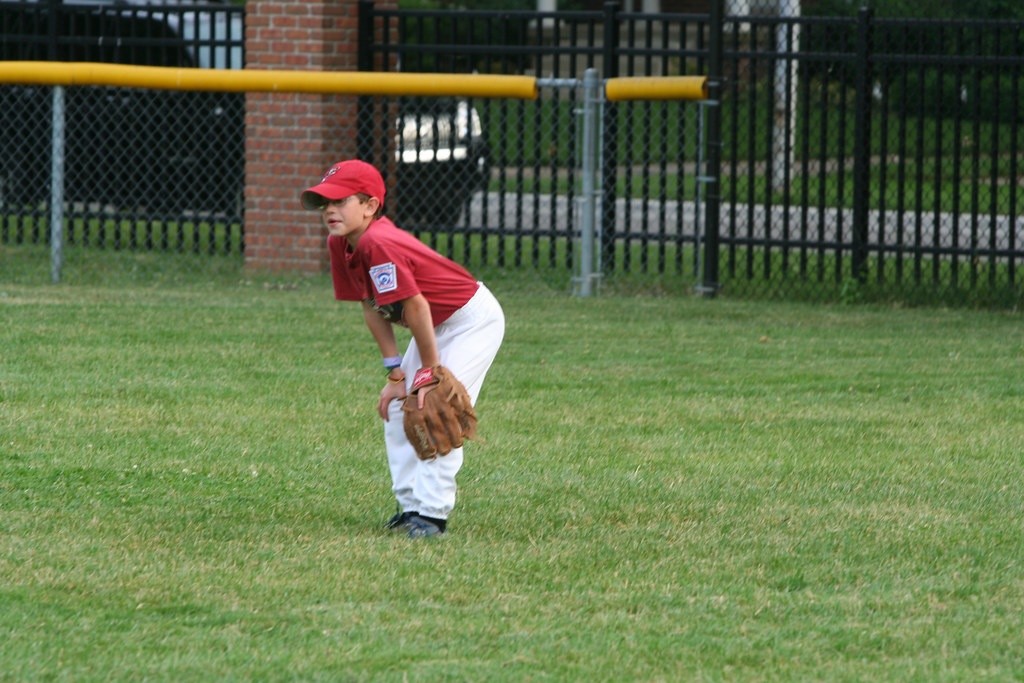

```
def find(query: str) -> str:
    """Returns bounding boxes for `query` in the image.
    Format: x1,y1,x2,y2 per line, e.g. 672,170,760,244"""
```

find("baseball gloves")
400,366,479,462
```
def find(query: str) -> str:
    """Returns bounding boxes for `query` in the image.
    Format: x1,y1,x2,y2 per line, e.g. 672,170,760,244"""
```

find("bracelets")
384,357,401,368
388,375,405,384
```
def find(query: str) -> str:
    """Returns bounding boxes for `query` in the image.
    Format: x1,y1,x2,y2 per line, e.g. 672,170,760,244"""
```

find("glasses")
316,194,370,210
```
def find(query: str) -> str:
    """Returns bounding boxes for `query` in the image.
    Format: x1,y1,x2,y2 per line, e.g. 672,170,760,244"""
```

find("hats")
300,159,385,213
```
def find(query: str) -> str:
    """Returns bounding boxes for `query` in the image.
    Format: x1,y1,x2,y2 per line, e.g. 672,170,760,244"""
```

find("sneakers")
405,516,447,540
383,511,419,531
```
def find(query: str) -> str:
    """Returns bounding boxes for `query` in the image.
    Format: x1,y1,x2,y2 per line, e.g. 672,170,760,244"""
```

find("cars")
1,4,493,231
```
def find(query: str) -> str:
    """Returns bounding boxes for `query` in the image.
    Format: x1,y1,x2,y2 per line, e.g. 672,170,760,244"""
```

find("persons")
300,160,507,537
